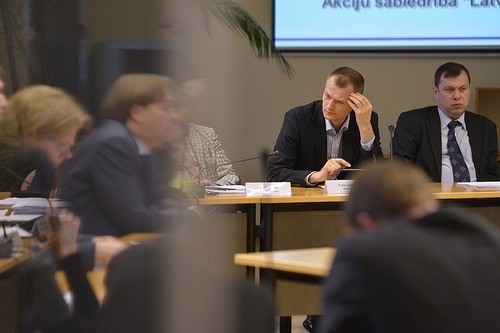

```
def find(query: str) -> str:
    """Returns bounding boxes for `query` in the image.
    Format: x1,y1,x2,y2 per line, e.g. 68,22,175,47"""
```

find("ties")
448,121,470,183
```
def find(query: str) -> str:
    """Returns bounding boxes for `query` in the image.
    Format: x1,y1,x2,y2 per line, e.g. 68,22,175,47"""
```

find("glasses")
49,138,74,152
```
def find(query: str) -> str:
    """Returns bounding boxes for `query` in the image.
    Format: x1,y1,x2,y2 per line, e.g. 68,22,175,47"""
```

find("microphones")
388,124,394,161
185,151,281,199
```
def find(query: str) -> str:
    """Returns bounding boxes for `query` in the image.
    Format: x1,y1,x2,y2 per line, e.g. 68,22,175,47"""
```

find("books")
457,182,500,190
204,185,245,194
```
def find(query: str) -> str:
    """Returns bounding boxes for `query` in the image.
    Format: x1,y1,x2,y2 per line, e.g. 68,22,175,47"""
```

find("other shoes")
303,315,320,333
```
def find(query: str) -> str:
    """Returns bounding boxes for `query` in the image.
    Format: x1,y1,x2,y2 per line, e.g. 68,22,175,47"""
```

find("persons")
267,66,384,333
0,213,275,333
390,61,500,182
0,74,239,237
320,160,500,333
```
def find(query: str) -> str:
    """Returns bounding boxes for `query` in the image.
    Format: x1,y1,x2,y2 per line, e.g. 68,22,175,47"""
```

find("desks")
0,181,500,333
